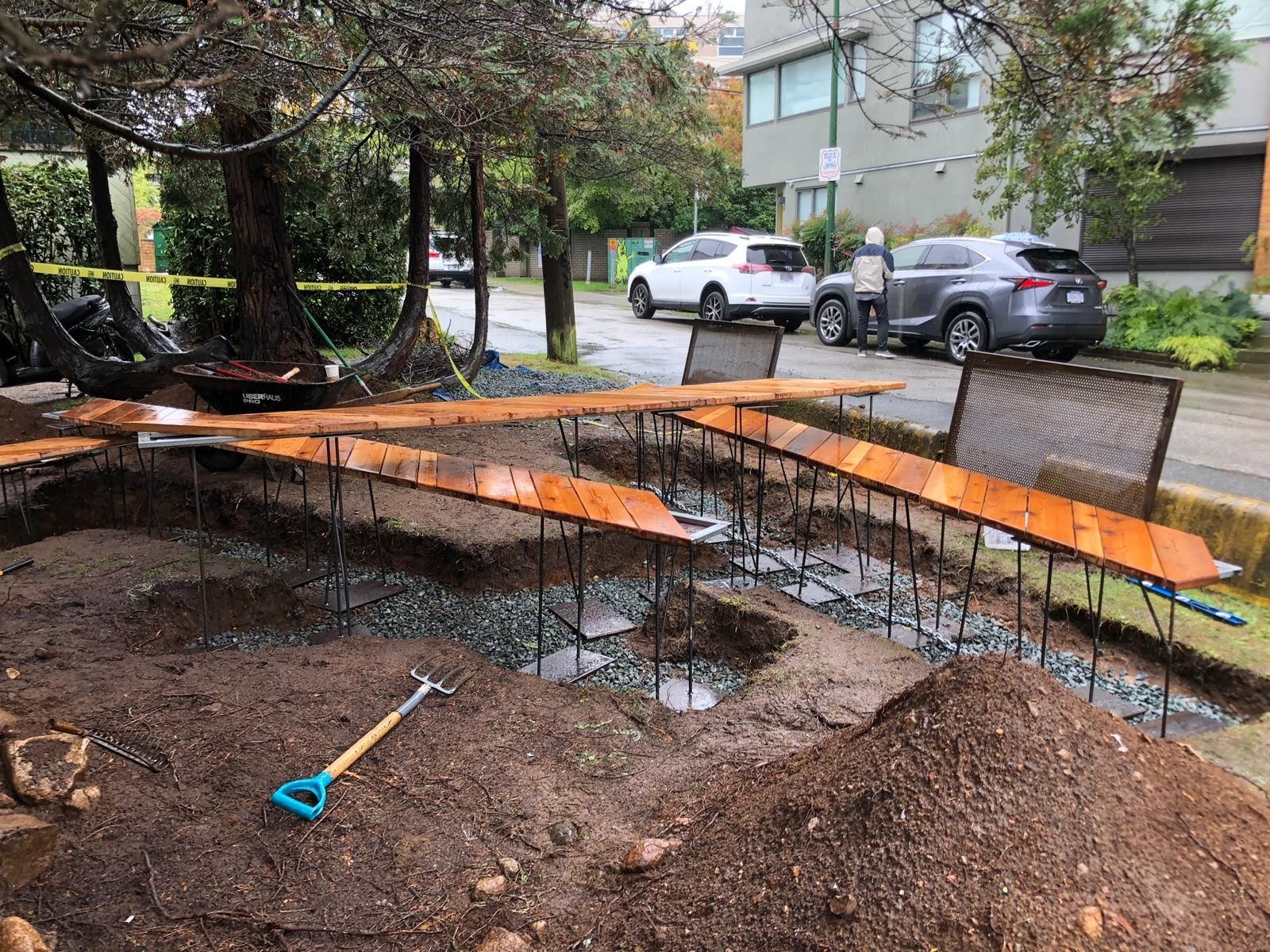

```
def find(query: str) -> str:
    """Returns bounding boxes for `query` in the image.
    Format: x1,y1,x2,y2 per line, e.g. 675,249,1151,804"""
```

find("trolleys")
173,359,444,474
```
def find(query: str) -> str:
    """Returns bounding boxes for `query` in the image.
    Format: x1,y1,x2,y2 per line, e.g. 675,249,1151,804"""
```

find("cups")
325,364,340,382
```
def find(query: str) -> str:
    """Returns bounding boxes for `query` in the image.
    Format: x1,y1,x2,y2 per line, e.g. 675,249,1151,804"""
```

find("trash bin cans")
152,221,176,273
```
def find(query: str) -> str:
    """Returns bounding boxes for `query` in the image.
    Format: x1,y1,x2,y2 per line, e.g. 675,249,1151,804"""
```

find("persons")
851,226,899,358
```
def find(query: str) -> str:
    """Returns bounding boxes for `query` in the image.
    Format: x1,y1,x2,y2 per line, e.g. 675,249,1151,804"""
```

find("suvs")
405,232,475,289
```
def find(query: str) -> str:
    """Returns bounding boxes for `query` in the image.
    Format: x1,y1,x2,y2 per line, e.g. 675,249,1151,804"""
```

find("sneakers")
857,349,867,356
875,350,897,358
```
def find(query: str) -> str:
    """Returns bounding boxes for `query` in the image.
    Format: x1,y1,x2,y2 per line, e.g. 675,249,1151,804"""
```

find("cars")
626,230,819,331
808,232,1109,366
